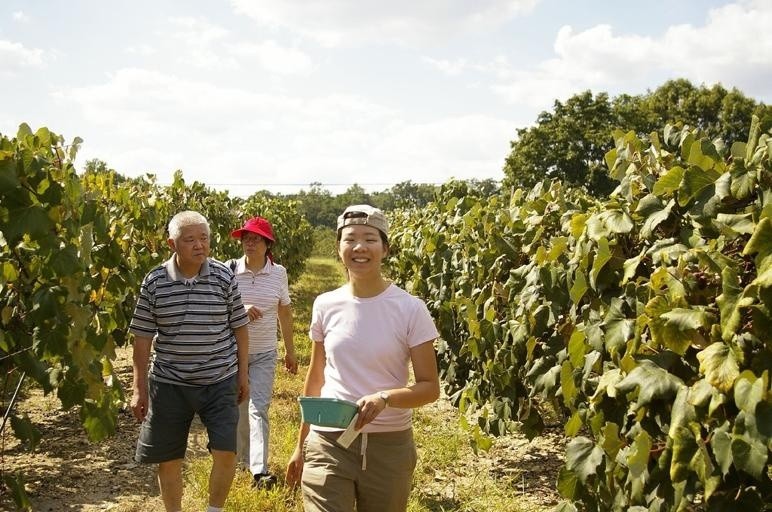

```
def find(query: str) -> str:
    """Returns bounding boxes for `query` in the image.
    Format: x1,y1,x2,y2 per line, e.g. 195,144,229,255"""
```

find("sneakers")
251,472,279,487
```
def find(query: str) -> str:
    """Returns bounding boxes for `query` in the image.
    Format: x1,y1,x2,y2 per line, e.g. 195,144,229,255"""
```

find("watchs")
378,391,388,406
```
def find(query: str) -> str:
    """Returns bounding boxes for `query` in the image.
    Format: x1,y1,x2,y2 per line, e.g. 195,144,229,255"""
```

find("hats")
231,217,276,244
336,205,388,239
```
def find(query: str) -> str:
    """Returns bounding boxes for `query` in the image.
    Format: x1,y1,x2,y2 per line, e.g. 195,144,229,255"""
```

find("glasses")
240,234,262,244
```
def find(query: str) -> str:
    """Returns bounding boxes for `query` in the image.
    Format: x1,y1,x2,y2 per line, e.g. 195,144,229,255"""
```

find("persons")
224,216,300,487
129,209,251,512
284,205,441,512
